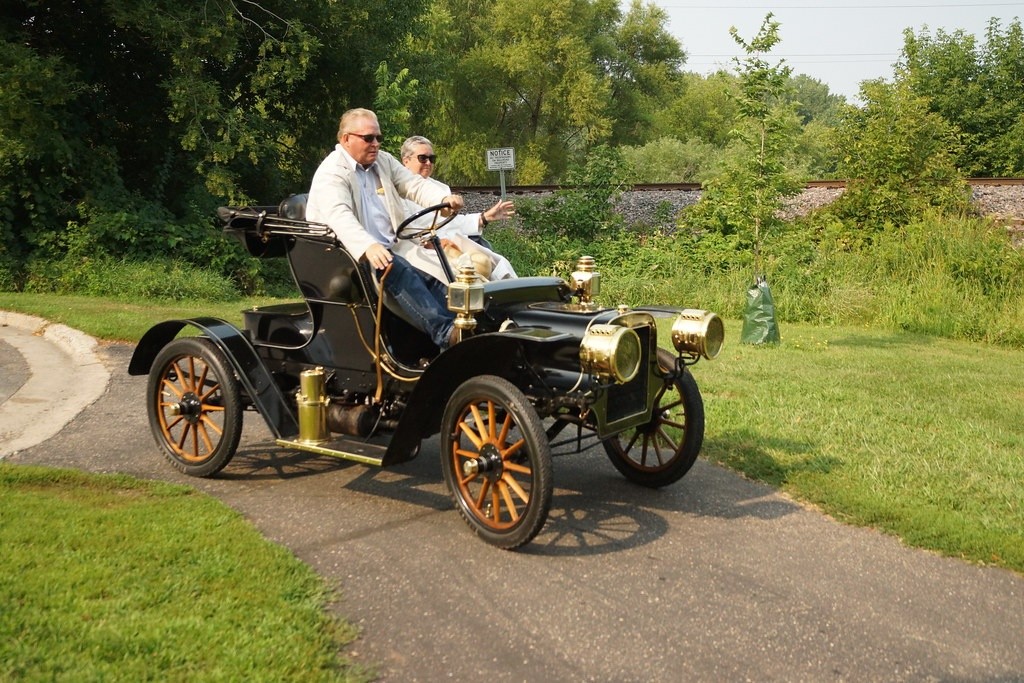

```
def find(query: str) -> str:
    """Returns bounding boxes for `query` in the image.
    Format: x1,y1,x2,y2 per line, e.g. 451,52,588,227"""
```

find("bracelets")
480,212,488,227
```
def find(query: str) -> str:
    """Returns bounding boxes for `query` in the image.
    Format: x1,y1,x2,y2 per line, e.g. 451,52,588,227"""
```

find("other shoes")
448,324,462,347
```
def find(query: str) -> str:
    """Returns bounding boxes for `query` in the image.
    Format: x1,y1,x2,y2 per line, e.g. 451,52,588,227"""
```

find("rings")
373,256,378,259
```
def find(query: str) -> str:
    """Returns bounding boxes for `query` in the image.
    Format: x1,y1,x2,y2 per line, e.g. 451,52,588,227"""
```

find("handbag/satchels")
443,244,491,277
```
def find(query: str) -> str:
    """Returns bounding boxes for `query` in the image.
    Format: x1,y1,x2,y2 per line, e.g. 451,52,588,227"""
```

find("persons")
391,136,519,332
307,108,474,351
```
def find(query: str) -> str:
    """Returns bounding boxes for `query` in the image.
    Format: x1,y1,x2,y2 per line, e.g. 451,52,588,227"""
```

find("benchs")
280,193,493,372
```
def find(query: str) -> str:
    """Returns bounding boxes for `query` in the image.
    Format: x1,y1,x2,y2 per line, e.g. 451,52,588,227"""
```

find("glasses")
348,133,385,143
407,154,437,163
